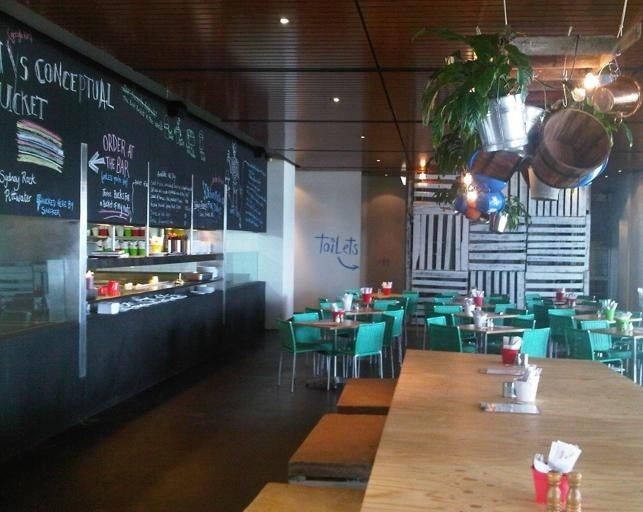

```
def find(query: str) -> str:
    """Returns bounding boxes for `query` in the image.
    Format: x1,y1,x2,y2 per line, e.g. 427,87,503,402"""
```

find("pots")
164,239,186,254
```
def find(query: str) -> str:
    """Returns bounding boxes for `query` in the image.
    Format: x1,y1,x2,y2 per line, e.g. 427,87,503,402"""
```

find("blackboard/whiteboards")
0,9,267,233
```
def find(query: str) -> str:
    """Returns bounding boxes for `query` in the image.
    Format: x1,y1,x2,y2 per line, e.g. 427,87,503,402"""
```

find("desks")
360,349,642,511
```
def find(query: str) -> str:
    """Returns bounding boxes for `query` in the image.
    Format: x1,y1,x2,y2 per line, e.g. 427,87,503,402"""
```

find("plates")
89,251,121,258
189,284,215,295
196,265,218,279
91,295,187,314
192,240,211,255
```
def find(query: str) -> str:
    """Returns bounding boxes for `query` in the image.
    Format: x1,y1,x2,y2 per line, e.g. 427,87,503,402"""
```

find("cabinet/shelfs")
86,250,224,313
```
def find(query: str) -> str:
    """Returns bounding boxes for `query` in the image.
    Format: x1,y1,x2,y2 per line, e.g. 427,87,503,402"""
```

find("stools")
235,483,365,512
287,412,384,482
335,375,395,414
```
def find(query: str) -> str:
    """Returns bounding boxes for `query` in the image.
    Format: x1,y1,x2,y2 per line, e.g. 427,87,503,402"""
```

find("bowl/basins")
149,243,163,254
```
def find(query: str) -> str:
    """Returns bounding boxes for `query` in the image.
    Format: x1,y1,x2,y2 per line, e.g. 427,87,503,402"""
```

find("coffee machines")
28,259,65,323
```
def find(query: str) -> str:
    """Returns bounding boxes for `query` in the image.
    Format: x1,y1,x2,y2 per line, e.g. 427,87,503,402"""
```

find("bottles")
503,382,514,398
545,469,562,511
565,470,581,511
521,353,528,366
513,353,521,365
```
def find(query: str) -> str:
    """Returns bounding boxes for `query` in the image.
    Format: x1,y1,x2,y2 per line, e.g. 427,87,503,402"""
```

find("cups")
98,225,108,237
138,227,144,236
604,308,614,321
500,347,519,367
531,462,570,504
382,288,391,296
556,292,563,301
340,299,352,311
332,311,345,322
124,227,131,236
115,240,145,257
91,227,98,236
473,313,486,328
116,227,124,237
616,320,629,330
132,227,138,236
362,293,373,303
567,299,576,307
473,296,483,306
464,304,475,317
107,280,120,296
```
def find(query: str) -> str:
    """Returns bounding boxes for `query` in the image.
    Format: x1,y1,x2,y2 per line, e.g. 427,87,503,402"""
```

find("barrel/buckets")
590,74,643,120
488,211,509,234
471,148,522,184
473,92,529,155
530,108,612,190
527,165,561,201
